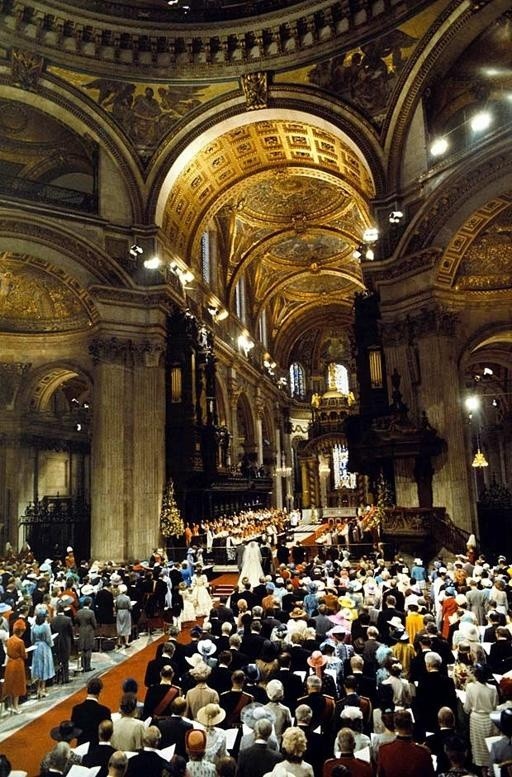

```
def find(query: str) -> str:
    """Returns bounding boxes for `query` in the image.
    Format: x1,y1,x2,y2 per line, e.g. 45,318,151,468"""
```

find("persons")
1,505,512,777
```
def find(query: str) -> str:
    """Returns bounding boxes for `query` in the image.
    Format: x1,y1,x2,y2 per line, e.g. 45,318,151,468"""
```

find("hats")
326,626,351,637
307,583,318,594
81,584,94,595
458,621,479,640
185,653,204,667
307,650,327,667
197,639,217,656
265,678,285,701
58,595,74,606
489,709,512,740
241,664,260,682
12,619,26,629
289,607,307,618
39,559,54,571
51,721,83,741
338,595,356,609
109,575,124,585
185,729,206,754
481,577,493,587
329,612,349,625
196,703,227,727
387,616,404,628
363,582,378,594
446,586,455,594
240,702,277,730
0,603,12,613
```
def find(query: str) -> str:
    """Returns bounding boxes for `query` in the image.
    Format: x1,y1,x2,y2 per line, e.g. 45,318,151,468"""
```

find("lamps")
129,245,143,256
389,211,403,223
472,416,489,467
208,306,219,316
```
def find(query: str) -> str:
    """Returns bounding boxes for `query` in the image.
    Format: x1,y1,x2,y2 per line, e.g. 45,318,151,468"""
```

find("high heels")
37,690,49,698
7,708,22,715
